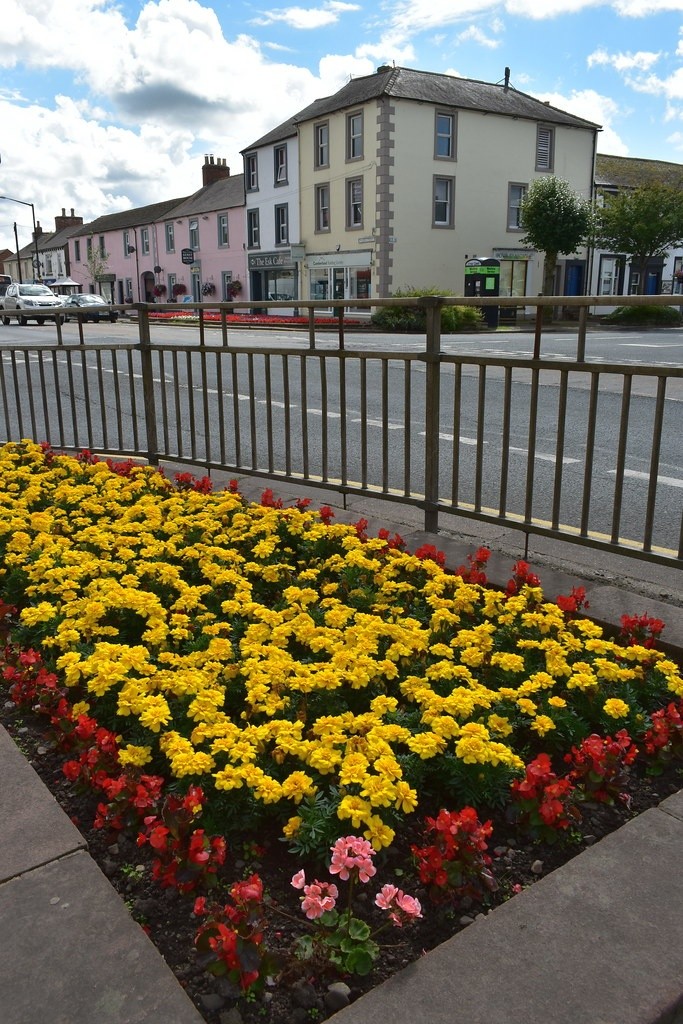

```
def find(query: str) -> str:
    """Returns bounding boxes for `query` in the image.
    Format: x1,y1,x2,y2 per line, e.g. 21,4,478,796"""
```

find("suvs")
5,283,65,325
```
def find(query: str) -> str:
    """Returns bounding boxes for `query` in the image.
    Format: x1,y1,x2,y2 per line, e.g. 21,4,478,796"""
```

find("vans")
0,274,13,325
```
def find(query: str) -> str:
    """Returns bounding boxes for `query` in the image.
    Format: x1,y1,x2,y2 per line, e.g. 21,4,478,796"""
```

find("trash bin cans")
500,305,517,326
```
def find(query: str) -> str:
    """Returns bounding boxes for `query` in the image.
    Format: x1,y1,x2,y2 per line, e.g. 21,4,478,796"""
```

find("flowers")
224,280,242,297
150,285,169,297
199,282,217,296
172,283,187,298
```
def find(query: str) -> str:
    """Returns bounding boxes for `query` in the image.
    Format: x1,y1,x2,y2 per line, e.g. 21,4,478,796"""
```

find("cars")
58,294,119,323
268,292,294,301
